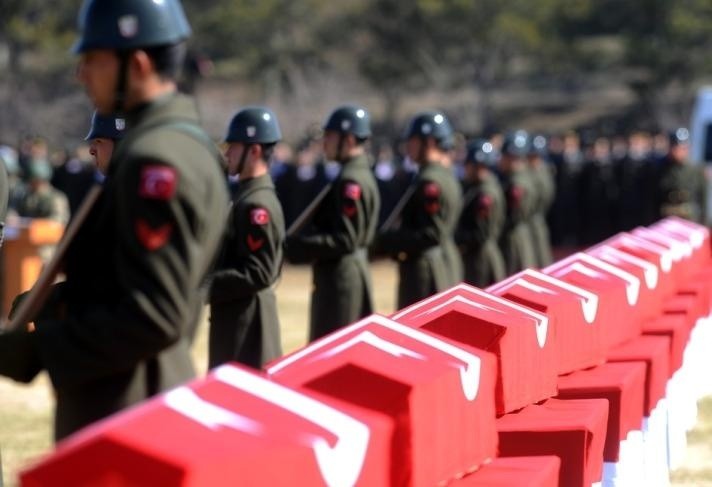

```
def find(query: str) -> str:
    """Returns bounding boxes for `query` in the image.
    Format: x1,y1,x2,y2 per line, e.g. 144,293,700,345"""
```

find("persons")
282,104,379,343
0,0,236,445
497,132,555,277
1,137,105,262
546,130,688,252
455,141,506,288
195,104,284,373
207,104,284,373
282,104,379,341
84,104,129,175
268,137,466,265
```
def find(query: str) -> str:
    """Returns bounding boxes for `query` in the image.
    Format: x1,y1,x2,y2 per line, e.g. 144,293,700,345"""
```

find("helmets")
321,104,373,140
400,110,453,149
69,0,193,55
225,107,282,144
84,107,126,141
669,127,694,153
468,129,549,165
